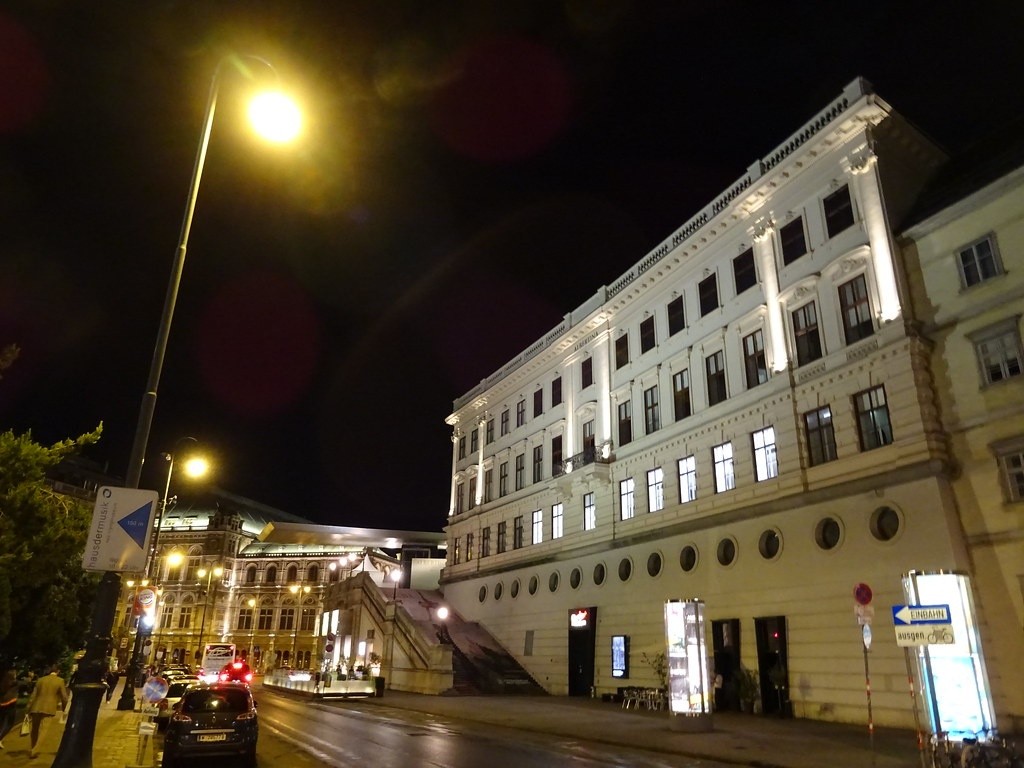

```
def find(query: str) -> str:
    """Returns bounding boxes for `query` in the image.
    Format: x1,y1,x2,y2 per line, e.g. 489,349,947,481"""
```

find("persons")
710,668,722,711
105,667,119,704
0,664,68,758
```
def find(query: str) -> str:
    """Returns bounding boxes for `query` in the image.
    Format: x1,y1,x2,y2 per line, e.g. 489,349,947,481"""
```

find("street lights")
290,579,313,668
195,557,224,666
116,435,208,710
133,538,188,689
53,52,305,768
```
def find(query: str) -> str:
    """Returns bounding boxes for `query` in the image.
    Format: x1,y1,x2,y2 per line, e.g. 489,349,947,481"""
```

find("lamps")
775,370,779,374
885,319,891,324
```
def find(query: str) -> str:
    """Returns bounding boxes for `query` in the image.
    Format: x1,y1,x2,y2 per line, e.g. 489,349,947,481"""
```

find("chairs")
622,685,668,710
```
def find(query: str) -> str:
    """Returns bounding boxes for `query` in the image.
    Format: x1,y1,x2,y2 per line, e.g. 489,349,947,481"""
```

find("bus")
199,642,236,672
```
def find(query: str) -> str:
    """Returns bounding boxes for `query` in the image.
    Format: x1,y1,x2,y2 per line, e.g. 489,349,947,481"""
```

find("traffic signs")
892,604,952,625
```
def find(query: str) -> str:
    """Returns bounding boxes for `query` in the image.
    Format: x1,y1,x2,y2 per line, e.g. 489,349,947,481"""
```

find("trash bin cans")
369,678,385,697
136,670,146,687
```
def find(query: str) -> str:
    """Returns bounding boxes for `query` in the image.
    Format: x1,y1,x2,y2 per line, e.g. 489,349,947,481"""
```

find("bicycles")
930,723,1024,768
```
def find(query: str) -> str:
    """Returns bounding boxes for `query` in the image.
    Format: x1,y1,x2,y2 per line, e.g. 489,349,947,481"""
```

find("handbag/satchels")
20,714,29,737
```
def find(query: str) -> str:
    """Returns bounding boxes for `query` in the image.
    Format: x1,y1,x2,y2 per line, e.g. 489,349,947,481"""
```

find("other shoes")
0,741,4,749
29,754,38,759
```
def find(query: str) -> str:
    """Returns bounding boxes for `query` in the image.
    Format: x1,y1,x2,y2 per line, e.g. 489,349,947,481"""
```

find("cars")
119,653,252,727
161,681,260,768
